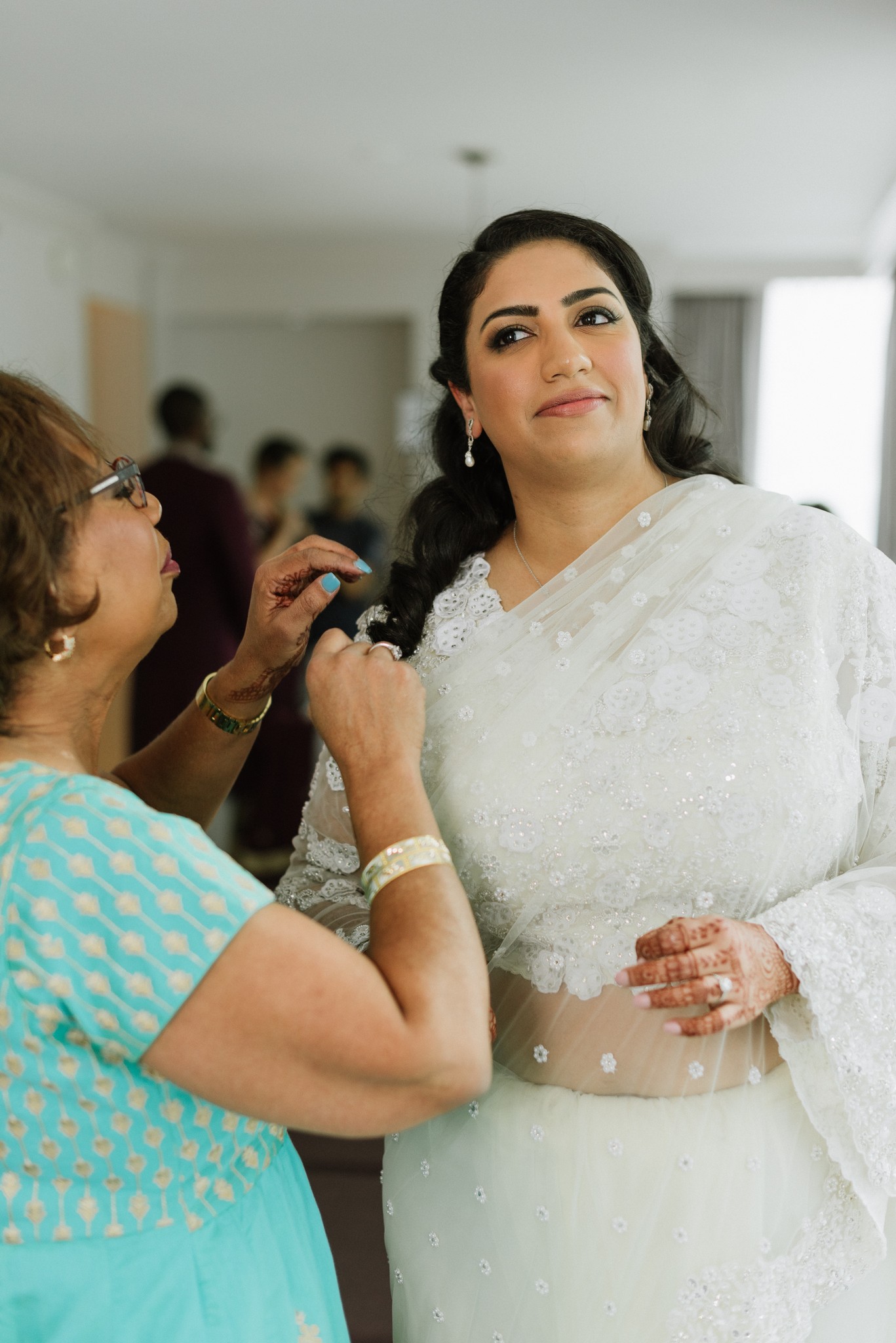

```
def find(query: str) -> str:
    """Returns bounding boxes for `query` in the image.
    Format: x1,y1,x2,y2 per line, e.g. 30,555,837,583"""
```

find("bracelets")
357,833,453,905
195,672,274,736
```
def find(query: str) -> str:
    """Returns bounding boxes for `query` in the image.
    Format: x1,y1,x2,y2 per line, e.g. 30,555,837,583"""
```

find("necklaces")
510,518,545,591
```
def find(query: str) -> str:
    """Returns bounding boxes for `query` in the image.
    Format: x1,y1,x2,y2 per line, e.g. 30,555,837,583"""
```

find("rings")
366,642,404,662
711,973,732,1006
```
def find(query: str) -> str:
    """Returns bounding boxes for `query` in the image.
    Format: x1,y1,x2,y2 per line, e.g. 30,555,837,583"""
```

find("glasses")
51,455,149,517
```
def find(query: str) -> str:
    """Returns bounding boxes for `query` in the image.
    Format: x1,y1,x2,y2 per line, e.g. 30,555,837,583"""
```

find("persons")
298,441,392,679
0,369,498,1343
118,381,251,769
273,205,895,1342
230,433,321,862
